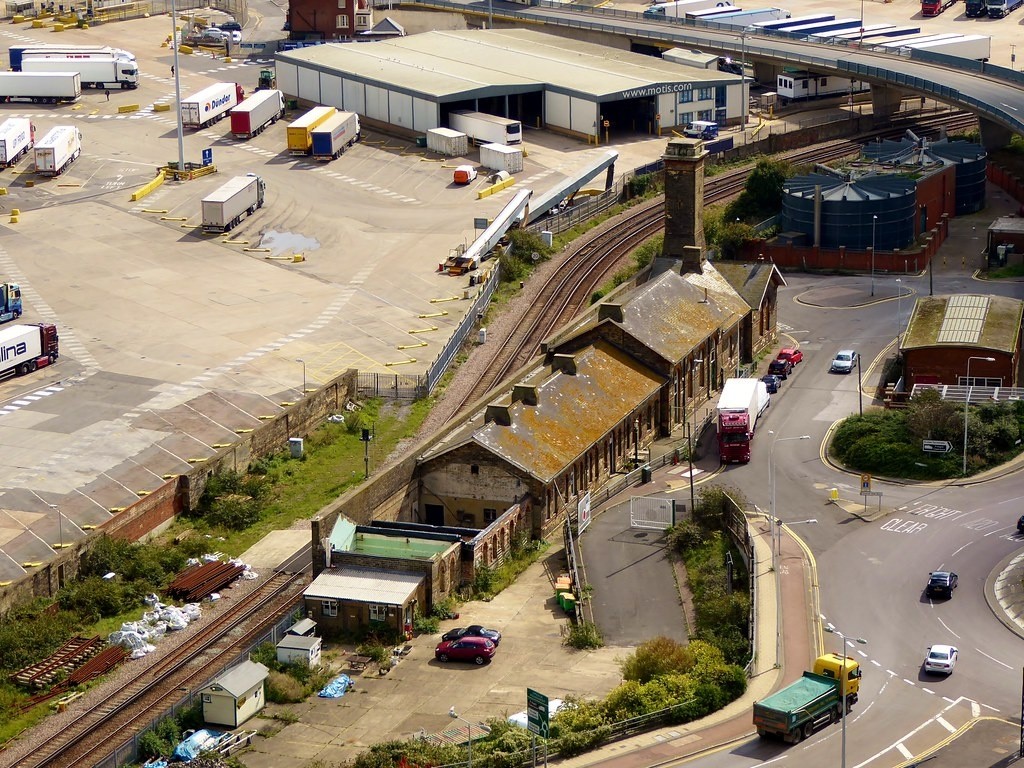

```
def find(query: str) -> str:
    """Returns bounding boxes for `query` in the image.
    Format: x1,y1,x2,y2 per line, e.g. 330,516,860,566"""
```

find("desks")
346,657,372,672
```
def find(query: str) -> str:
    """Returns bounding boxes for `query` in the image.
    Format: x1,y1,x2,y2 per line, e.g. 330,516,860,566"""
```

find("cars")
1017,516,1024,535
442,625,502,647
923,644,959,676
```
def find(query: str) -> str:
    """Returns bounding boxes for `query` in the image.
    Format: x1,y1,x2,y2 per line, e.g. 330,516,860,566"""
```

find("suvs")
760,348,803,394
435,637,497,666
831,350,857,374
207,21,242,43
926,570,958,601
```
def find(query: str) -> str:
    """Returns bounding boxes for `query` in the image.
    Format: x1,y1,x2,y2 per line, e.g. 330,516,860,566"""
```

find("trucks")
752,653,862,743
448,109,522,146
643,0,991,104
718,378,771,465
683,120,719,140
454,165,478,185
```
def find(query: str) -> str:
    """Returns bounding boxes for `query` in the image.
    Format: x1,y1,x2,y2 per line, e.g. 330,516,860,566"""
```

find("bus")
6,0,35,18
96,3,135,17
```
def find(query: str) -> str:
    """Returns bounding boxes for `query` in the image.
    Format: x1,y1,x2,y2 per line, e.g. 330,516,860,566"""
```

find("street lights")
962,355,995,477
448,711,492,768
767,431,819,570
824,626,867,768
871,215,877,297
692,358,703,441
896,278,901,356
734,24,763,131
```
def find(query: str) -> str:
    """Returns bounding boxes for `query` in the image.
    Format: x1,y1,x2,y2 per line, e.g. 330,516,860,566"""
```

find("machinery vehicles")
255,68,276,87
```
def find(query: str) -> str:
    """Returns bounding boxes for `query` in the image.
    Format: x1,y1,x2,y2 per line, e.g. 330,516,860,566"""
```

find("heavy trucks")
0,283,58,386
0,83,361,234
921,0,1024,21
0,44,140,104
